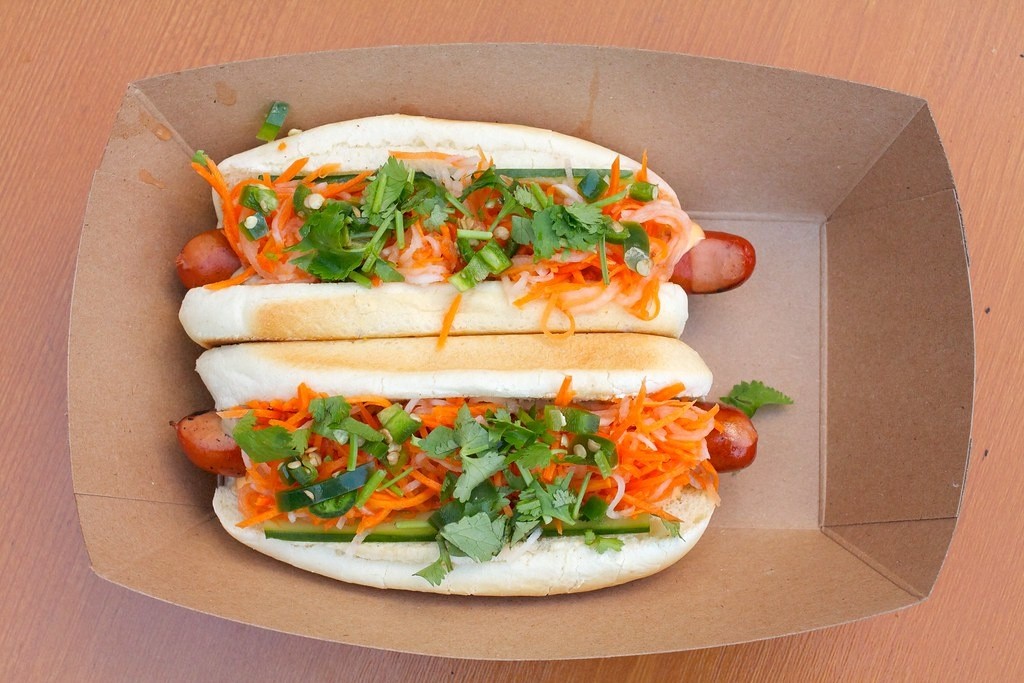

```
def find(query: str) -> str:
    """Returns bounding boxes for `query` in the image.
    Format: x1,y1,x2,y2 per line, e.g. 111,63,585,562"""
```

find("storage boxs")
66,43,973,662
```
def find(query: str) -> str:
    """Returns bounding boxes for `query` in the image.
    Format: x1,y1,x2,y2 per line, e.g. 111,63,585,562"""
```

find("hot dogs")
166,112,757,348
176,333,791,597
